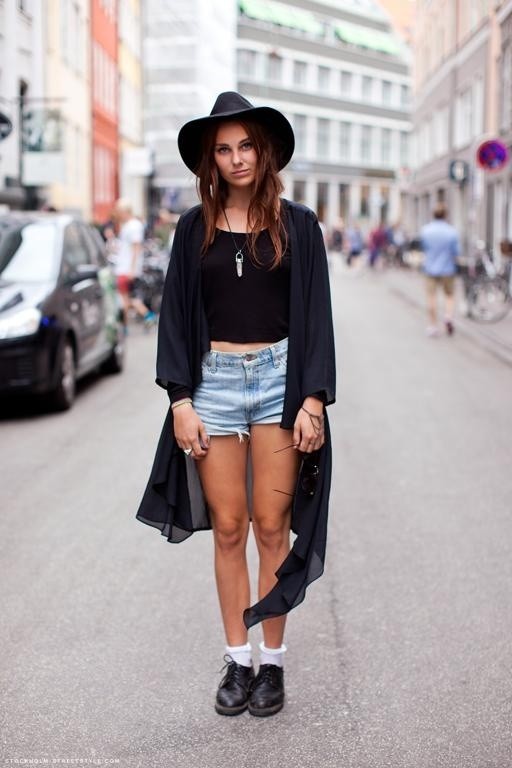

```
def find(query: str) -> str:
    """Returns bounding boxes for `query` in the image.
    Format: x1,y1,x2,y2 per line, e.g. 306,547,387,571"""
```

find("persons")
150,207,176,247
384,218,409,268
346,219,363,266
418,201,460,338
135,90,337,717
369,217,387,270
102,199,155,328
333,225,343,251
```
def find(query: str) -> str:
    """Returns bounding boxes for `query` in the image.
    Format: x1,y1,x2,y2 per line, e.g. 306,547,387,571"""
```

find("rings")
183,448,193,456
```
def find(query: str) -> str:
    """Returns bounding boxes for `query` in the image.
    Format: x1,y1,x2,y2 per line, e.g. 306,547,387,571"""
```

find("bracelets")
172,399,193,409
301,406,327,437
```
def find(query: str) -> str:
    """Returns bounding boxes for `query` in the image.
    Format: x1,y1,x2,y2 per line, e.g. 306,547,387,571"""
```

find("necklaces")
219,200,260,278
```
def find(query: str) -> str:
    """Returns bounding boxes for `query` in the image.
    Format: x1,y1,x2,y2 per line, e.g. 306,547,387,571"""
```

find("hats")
178,91,296,171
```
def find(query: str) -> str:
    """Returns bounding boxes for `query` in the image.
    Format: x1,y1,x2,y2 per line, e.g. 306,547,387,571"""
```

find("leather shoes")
247,663,285,716
214,658,253,716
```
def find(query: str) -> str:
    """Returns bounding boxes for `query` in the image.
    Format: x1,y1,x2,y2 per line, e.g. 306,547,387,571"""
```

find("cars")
0,205,127,409
